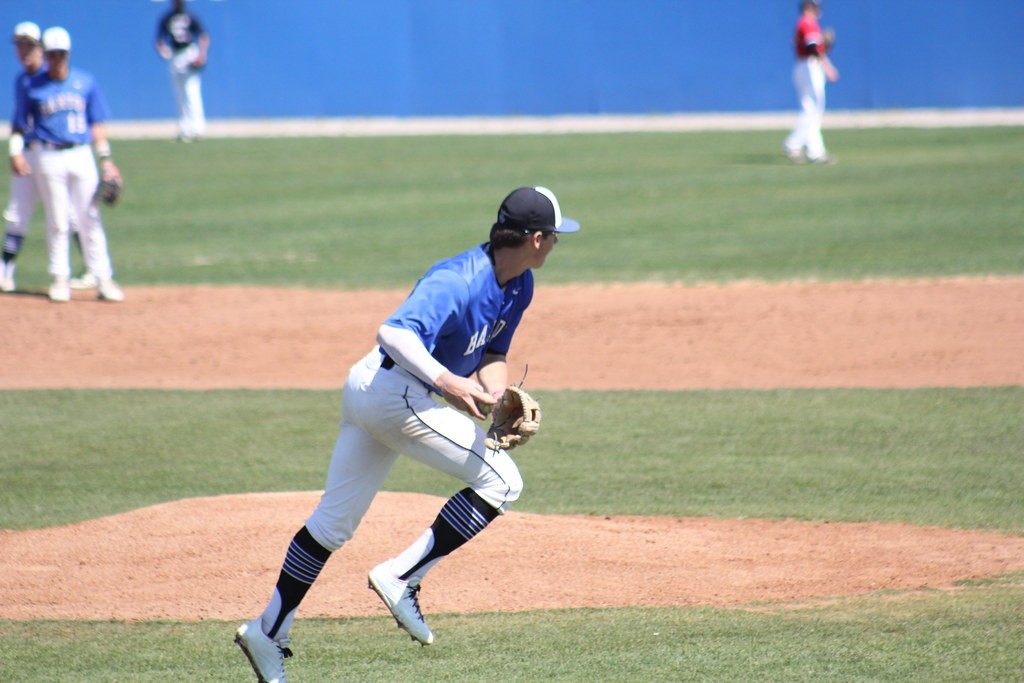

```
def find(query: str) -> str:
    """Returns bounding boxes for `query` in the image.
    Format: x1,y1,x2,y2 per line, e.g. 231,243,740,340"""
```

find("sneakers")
235,617,293,683
367,558,434,647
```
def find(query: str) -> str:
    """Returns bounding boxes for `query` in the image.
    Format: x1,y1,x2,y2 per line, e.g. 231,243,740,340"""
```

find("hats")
498,186,580,232
14,22,41,45
44,27,70,51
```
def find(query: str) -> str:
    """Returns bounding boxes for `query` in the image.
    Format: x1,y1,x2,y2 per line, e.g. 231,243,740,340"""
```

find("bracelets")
95,138,110,156
9,134,24,156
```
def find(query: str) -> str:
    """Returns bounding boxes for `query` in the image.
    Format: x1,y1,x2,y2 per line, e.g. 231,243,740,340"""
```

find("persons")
783,0,839,163
155,0,208,143
0,21,124,302
234,186,582,683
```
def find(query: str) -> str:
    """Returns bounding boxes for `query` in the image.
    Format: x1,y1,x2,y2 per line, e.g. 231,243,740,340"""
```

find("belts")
41,140,72,150
380,357,394,370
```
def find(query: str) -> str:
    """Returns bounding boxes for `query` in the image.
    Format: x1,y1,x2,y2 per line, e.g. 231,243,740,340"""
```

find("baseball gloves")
99,179,122,206
484,386,541,450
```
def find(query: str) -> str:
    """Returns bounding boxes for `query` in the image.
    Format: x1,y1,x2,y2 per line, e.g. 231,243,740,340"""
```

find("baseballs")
478,402,494,414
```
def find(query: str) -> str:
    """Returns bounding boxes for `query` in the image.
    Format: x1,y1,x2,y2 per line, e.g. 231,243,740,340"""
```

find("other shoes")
0,260,15,291
69,273,97,289
98,286,124,300
48,285,70,301
